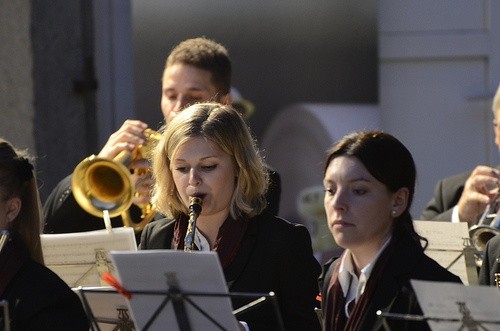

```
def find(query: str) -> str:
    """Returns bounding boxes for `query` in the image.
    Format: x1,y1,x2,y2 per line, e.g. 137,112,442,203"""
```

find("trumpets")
71,128,163,233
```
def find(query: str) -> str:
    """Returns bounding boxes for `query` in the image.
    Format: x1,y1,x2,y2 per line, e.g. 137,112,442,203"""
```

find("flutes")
183,195,203,251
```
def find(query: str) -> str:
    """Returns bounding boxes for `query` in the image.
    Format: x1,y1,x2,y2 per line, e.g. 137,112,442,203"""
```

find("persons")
314,130,464,331
0,137,89,331
477,233,500,287
136,102,322,331
40,37,284,246
420,83,500,286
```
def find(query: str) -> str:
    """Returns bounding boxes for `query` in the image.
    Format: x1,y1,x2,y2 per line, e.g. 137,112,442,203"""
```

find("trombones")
468,185,500,266
